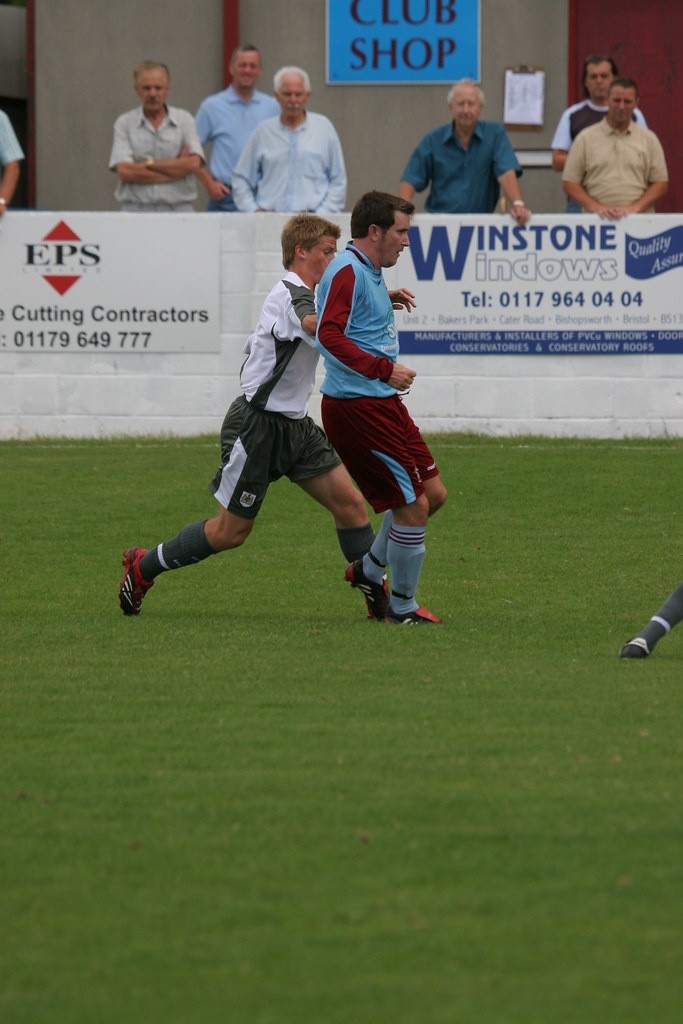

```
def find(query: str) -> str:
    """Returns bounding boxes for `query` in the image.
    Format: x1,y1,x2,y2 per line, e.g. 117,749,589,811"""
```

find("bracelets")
513,200,525,206
0,198,8,206
145,160,154,169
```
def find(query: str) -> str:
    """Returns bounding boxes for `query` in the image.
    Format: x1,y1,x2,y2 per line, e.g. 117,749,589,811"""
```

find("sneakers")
621,636,650,659
118,548,155,617
387,607,447,625
343,559,391,620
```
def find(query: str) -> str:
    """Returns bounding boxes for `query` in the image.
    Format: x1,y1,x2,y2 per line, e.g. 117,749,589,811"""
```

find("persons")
109,60,207,212
0,108,25,216
620,582,683,658
230,66,348,214
316,190,448,624
193,45,281,213
119,213,417,615
561,78,669,221
397,78,531,226
549,54,649,213
